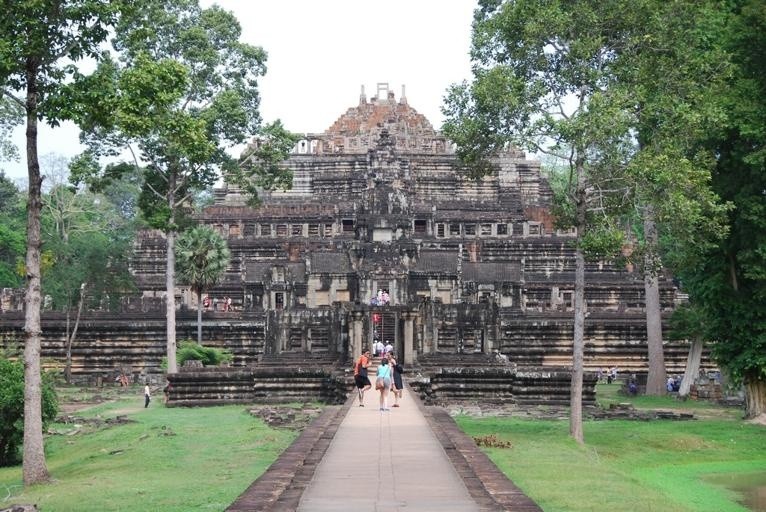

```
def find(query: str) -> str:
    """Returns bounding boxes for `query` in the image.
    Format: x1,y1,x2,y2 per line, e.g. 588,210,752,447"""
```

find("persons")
353,329,404,411
203,295,232,312
607,366,680,395
378,289,390,305
143,382,174,408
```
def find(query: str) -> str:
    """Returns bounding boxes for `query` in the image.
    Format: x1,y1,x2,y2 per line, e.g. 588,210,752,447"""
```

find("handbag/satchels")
375,377,385,390
358,367,368,378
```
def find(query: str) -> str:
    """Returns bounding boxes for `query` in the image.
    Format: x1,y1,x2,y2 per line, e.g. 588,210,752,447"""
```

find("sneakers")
359,404,400,411
